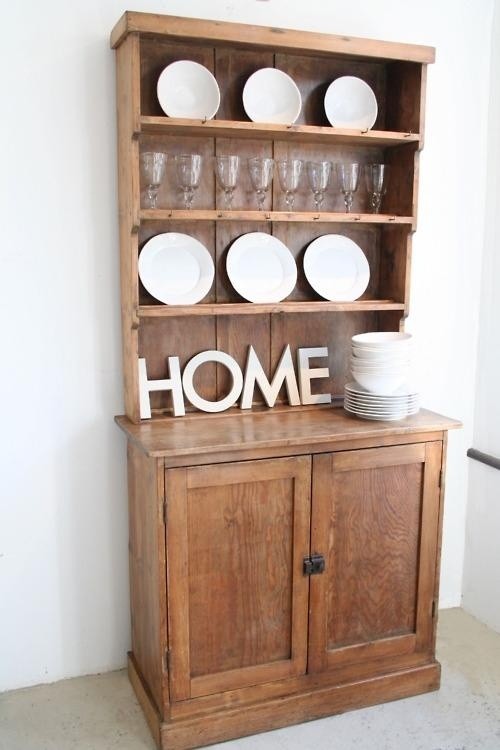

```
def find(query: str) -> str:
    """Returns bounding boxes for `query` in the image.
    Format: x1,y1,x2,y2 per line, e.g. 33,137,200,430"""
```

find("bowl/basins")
349,332,416,394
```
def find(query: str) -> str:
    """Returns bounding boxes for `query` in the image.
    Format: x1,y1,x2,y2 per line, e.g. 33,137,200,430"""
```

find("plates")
138,232,215,306
342,381,423,422
157,59,221,122
241,67,302,125
322,75,378,130
303,232,371,303
226,231,298,304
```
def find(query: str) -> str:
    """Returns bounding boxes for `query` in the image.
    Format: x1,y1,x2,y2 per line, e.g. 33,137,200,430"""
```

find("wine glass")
140,152,169,210
335,161,361,213
277,159,304,211
363,163,392,214
212,154,241,210
247,156,277,210
171,154,203,210
306,160,333,212
